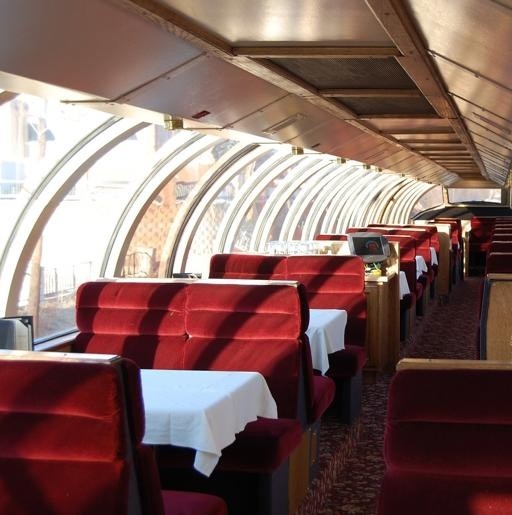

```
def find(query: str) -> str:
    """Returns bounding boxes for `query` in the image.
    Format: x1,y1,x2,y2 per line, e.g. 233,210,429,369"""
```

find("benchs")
383,357,512,515
4,350,224,514
207,219,512,422
70,278,304,511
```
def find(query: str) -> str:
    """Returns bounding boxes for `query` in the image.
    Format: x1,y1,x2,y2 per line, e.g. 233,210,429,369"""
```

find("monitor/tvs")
346,231,391,263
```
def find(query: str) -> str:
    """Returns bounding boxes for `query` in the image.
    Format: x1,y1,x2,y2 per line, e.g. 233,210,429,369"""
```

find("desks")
137,368,280,513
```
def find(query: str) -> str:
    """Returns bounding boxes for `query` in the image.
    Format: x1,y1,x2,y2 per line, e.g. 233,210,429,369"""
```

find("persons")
365,240,380,255
